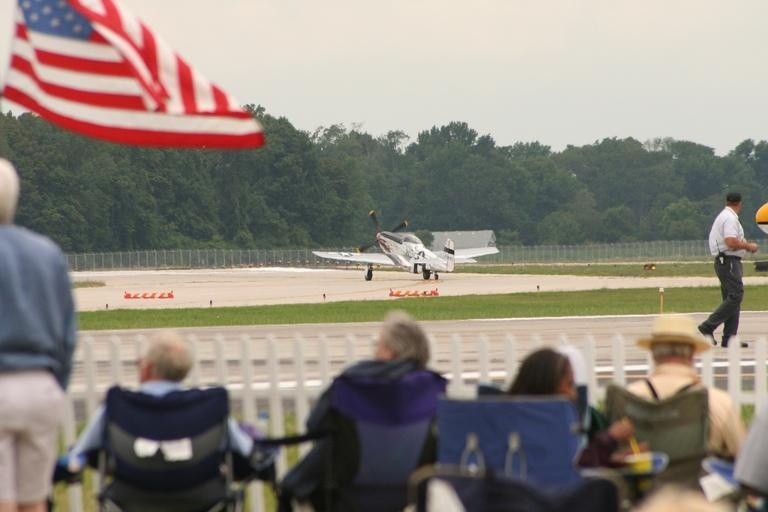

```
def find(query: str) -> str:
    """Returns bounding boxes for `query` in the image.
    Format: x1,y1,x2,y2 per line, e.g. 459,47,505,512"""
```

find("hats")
635,315,713,352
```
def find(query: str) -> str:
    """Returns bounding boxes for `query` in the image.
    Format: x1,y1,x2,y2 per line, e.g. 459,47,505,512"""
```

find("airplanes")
311,210,500,280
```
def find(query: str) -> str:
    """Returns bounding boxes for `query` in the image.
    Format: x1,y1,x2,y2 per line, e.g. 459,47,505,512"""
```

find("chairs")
436,396,617,512
606,384,708,466
288,373,447,511
52,386,276,511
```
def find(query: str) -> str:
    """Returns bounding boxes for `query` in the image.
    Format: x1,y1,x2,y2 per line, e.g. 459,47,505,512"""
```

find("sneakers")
721,336,748,348
698,323,717,346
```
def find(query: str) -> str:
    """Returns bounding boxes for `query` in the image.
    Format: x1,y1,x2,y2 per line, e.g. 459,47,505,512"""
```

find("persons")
68,327,263,512
696,192,760,348
273,309,446,510
0,158,78,511
626,313,744,490
503,348,639,510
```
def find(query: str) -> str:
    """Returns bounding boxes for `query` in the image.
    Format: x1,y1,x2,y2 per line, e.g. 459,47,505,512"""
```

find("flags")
1,0,269,150
